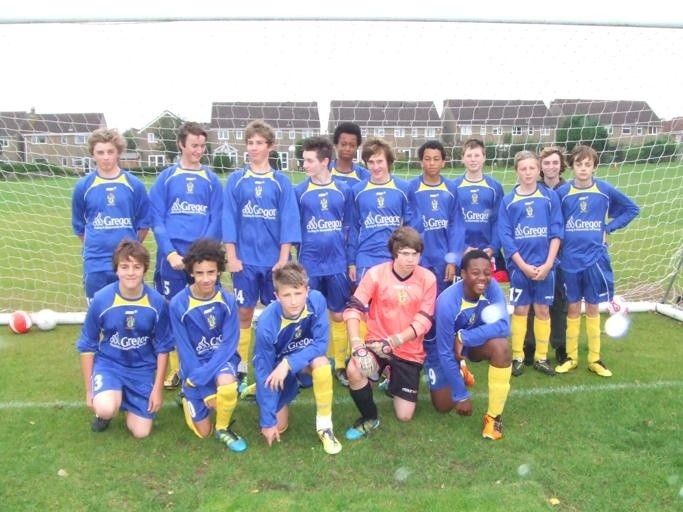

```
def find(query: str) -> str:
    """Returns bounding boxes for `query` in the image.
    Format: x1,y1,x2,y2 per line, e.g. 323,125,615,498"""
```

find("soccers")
10,311,31,334
609,295,628,313
36,308,57,328
605,316,627,336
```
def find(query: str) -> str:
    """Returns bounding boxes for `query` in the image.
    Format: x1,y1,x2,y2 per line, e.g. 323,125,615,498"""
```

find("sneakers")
332,367,351,388
554,356,580,376
162,368,181,391
533,360,558,377
238,372,257,400
460,359,474,387
588,360,614,378
90,414,112,432
316,426,343,456
344,415,381,442
482,415,504,440
213,419,248,452
512,359,526,378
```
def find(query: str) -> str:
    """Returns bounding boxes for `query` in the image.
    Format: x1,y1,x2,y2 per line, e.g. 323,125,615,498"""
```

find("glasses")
396,251,421,258
